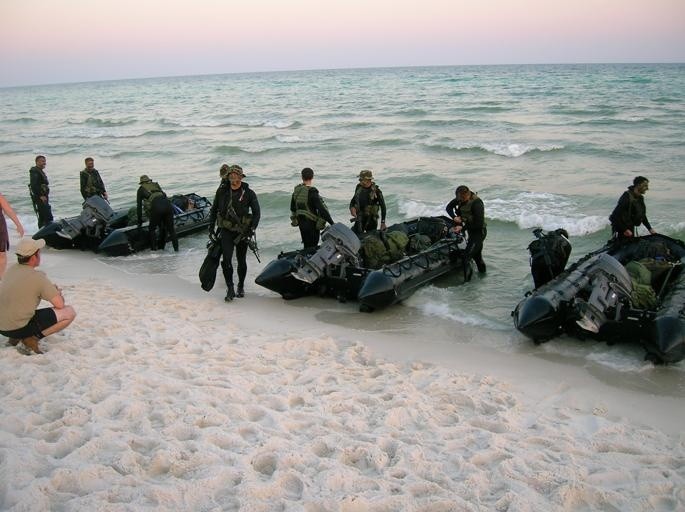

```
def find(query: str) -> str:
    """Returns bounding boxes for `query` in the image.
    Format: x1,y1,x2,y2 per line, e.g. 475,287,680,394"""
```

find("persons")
0,235,76,356
445,184,488,281
290,167,335,250
607,175,658,239
347,169,388,241
207,165,260,301
218,164,231,185
0,192,25,287
528,228,572,291
137,174,178,252
28,155,56,227
79,157,108,203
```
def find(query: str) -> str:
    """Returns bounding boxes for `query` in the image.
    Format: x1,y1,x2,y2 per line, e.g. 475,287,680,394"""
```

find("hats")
359,170,375,180
220,165,245,178
138,175,152,185
16,238,46,258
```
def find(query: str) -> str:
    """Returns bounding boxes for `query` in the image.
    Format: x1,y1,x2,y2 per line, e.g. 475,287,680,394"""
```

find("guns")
533,228,555,276
351,211,364,234
28,185,39,219
453,206,467,244
228,205,261,265
94,181,111,205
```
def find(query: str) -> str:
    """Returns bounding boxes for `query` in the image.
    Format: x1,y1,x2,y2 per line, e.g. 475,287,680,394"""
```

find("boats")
253,215,466,312
31,192,211,256
512,233,684,367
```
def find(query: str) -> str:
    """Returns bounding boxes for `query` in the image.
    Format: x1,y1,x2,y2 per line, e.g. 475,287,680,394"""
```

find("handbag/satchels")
635,239,669,259
416,216,447,241
359,229,409,264
408,233,431,251
127,205,147,225
624,258,670,307
171,194,188,211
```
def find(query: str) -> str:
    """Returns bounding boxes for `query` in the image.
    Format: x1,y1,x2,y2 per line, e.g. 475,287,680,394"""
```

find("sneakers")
16,339,44,355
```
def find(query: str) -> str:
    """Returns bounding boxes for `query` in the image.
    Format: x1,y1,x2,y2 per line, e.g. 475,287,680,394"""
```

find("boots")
237,282,245,298
225,284,235,301
